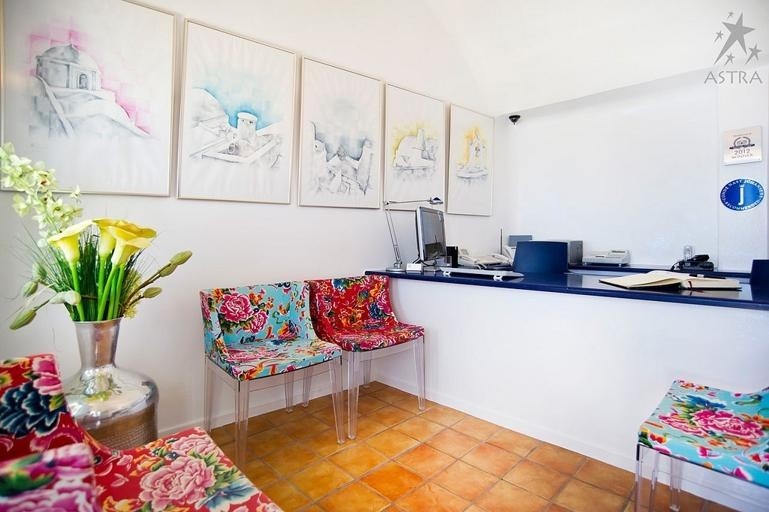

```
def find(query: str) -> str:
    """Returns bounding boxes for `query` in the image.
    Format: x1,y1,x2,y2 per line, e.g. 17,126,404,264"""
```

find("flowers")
0,142,193,330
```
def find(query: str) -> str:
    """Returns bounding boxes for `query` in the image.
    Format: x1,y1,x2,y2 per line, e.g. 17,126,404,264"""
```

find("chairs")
199,281,346,472
303,275,426,441
633,377,769,512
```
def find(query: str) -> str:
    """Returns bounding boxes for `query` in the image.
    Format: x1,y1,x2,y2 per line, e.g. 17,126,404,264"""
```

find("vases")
61,319,158,450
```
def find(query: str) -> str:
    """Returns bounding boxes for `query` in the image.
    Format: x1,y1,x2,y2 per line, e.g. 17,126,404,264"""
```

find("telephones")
460,252,513,266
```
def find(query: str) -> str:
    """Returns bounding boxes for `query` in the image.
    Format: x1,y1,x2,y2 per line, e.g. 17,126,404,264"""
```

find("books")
599,270,742,289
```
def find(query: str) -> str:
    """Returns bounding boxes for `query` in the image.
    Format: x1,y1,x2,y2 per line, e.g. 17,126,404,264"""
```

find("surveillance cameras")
508,115,521,124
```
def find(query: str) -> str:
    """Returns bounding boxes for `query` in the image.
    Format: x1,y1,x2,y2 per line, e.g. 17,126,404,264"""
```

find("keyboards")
442,267,524,282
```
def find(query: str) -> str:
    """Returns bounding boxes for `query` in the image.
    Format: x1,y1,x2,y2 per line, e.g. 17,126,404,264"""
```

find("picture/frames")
2,1,177,196
177,19,298,205
446,103,494,217
298,56,384,208
382,83,446,212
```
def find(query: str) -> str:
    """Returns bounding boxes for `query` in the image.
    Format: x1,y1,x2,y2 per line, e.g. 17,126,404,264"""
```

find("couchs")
0,354,286,512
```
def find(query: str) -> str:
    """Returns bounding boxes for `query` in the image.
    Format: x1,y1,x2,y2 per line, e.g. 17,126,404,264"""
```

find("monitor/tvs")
411,206,447,269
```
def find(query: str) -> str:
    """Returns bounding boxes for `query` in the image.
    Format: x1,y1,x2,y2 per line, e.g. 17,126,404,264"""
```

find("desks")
364,263,769,512
